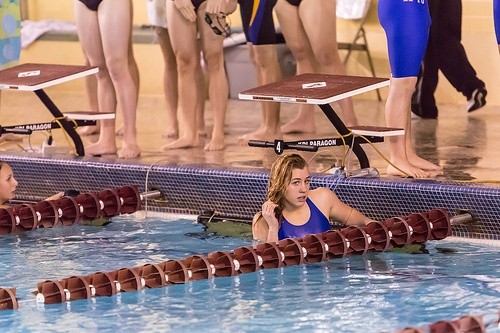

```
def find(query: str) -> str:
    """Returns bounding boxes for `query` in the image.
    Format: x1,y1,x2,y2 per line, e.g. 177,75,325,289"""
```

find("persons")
79,0,139,136
203,0,237,132
0,161,64,207
236,0,283,143
377,0,442,178
251,153,379,244
164,0,228,152
71,0,141,159
493,0,500,54
411,0,488,120
274,0,359,133
146,0,209,137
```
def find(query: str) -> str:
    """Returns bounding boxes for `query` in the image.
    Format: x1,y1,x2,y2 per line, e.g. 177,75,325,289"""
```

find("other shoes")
467,83,487,113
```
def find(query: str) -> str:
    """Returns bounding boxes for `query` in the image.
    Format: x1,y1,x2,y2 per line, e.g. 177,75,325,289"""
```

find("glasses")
204,12,231,38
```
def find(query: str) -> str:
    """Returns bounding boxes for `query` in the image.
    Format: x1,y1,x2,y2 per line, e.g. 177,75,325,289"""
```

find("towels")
335,0,366,21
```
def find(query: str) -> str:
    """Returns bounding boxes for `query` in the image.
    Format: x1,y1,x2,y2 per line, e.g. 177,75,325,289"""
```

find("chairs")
336,0,382,101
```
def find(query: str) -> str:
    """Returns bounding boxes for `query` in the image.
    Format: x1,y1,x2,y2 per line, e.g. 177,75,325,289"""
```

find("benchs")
20,19,161,46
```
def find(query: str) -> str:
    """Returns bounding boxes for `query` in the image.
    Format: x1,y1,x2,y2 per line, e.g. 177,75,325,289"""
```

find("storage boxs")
222,41,297,101
